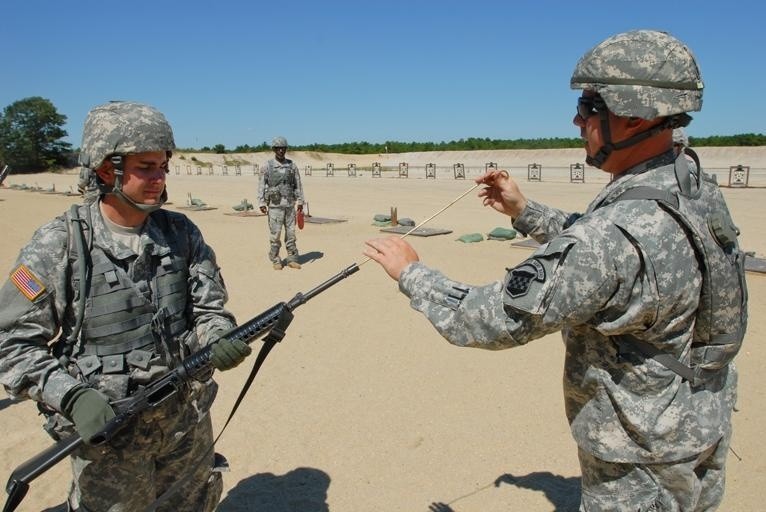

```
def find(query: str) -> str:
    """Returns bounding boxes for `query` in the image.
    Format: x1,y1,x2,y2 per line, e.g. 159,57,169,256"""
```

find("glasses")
576,96,606,117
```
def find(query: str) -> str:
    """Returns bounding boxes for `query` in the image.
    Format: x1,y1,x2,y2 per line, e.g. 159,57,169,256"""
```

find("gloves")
297,205,304,212
64,388,130,451
208,338,252,372
259,206,267,213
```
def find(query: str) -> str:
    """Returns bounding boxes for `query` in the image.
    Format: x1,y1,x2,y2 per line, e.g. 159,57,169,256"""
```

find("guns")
6,262,359,495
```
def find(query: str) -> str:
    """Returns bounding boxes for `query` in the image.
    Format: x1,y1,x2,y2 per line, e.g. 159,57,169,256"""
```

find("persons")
77,167,98,197
0,100,252,512
257,136,305,270
362,30,748,512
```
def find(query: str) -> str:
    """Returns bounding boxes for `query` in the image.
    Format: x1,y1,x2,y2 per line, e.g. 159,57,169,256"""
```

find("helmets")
77,100,176,170
570,30,704,120
271,137,288,148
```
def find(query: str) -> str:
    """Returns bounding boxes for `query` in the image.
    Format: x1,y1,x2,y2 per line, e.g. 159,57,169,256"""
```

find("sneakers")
288,261,301,268
273,261,282,270
